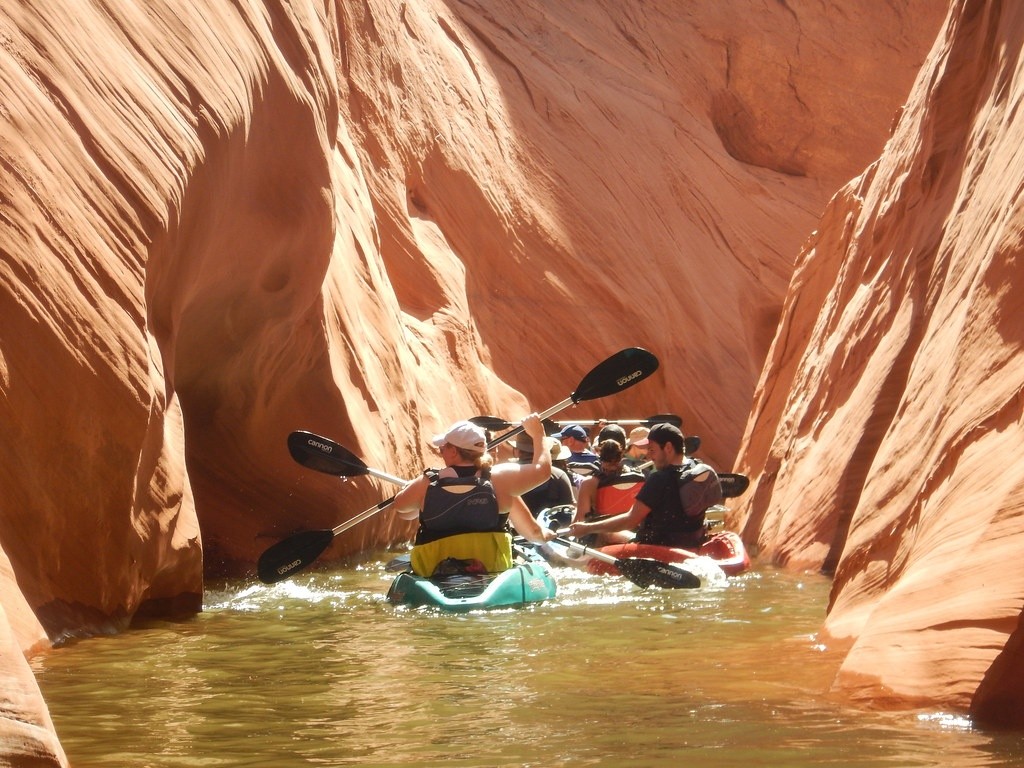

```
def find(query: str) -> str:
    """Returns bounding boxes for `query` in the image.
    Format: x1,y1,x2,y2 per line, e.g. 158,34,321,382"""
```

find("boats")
388,545,557,614
536,502,751,578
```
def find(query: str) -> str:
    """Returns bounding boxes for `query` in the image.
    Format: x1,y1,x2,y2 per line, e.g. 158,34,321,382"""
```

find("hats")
505,423,554,453
625,426,650,445
432,420,487,452
647,422,686,447
551,424,587,438
546,437,572,460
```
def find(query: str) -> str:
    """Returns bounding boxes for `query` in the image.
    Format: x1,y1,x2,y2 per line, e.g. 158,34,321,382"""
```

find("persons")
394,414,557,576
506,410,726,551
574,438,625,523
552,423,598,463
626,427,653,475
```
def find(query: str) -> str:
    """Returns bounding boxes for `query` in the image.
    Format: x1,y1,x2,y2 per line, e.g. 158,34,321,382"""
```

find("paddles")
467,414,685,432
256,347,660,586
286,428,702,591
632,435,750,499
